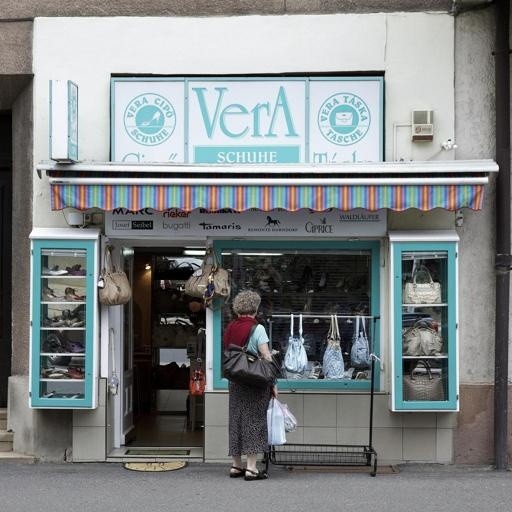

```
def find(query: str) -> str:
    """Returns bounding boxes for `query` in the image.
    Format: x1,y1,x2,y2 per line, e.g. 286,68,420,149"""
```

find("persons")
222,290,278,480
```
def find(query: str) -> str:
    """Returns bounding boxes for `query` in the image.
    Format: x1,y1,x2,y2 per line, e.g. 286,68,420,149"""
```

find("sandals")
41,263,86,275
228,466,243,478
41,364,85,379
43,310,84,328
42,340,86,354
243,468,268,481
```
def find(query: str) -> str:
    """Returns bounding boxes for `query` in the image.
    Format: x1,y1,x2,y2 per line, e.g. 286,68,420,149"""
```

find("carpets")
122,447,192,473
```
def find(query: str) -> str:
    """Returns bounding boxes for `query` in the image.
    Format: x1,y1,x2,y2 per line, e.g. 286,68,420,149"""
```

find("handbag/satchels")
402,327,443,356
107,374,120,396
404,281,443,305
220,342,278,389
284,337,309,375
321,338,344,380
184,264,232,307
97,270,132,305
189,369,205,397
168,261,195,279
153,322,197,347
349,331,373,369
403,372,446,401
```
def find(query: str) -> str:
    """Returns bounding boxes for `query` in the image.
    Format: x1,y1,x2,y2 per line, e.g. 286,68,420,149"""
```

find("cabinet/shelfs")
28,232,100,411
387,236,461,414
151,246,211,429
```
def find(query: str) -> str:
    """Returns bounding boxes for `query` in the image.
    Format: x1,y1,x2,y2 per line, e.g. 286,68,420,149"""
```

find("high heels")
41,287,84,302
